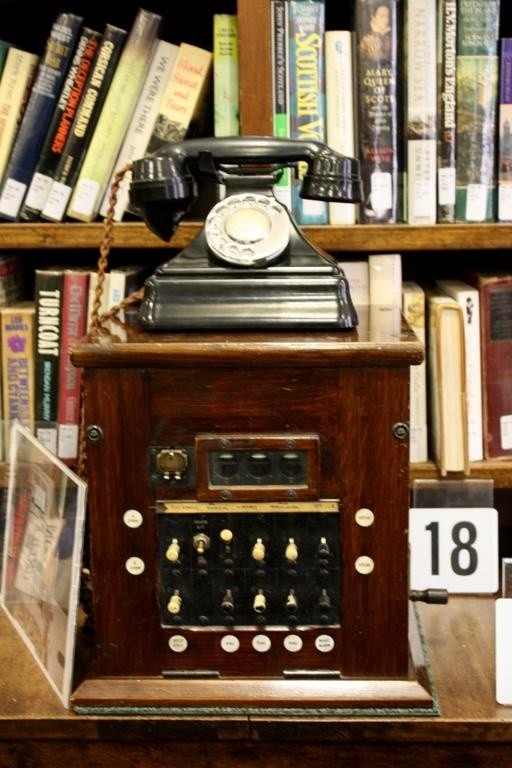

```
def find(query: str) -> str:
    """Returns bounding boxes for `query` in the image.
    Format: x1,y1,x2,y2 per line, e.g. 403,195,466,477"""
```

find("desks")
0,594,512,768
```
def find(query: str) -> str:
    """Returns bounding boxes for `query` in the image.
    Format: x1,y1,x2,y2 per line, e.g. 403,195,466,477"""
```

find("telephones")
127,135,370,331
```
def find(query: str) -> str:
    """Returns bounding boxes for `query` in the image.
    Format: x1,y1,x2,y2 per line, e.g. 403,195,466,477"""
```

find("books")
337,254,512,479
1,2,512,225
2,251,152,463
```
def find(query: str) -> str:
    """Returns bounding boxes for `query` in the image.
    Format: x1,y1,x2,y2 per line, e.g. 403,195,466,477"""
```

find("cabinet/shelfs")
0,1,512,490
